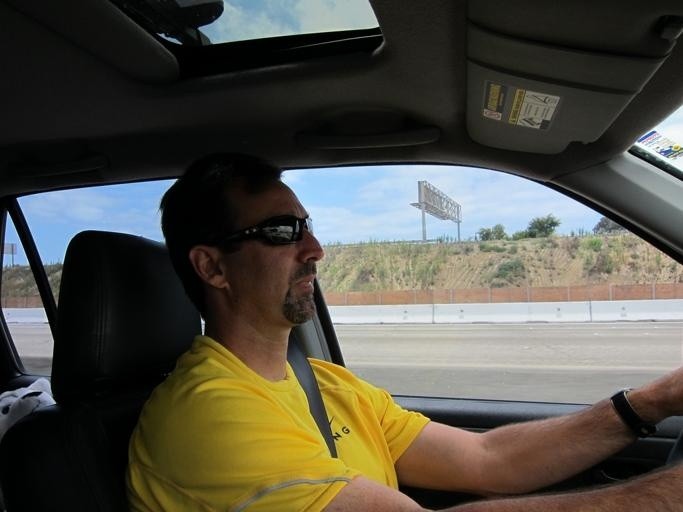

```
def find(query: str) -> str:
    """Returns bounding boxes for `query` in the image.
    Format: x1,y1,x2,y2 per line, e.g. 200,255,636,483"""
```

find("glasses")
207,215,314,247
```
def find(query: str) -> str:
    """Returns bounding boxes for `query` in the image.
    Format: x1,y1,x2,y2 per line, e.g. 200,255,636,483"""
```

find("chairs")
1,229,203,512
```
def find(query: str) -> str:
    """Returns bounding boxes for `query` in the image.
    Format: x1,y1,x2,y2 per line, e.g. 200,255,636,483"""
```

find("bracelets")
610,389,658,438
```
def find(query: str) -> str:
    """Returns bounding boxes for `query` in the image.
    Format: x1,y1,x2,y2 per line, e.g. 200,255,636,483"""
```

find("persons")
124,152,683,512
